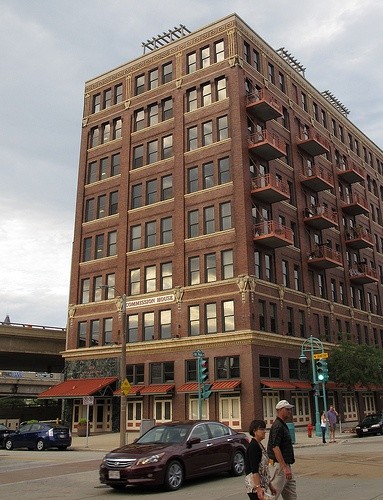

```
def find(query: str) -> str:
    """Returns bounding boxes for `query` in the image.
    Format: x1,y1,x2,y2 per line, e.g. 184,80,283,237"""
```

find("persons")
267,400,297,500
285,415,290,423
320,410,328,443
326,406,338,443
244,420,278,500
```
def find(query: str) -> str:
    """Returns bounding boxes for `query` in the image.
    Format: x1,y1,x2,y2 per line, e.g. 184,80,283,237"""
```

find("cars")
0,423,11,448
98,419,252,492
4,422,72,451
356,414,383,438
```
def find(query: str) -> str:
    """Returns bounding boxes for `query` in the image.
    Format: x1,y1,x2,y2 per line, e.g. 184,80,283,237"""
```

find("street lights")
297,335,324,437
98,284,128,447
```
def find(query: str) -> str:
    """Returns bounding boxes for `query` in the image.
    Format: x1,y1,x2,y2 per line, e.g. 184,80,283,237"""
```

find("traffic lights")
199,360,208,384
311,383,320,396
316,361,325,382
202,383,213,400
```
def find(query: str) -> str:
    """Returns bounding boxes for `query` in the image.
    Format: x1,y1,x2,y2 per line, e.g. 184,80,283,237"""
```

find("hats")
276,400,295,409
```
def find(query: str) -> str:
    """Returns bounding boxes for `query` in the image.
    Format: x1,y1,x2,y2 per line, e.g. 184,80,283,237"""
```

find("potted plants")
78,418,90,437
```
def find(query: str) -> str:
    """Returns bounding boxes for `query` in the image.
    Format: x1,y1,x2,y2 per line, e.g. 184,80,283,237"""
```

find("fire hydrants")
307,422,314,438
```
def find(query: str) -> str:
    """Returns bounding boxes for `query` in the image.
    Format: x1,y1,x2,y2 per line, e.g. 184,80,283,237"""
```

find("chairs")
168,430,181,442
194,427,205,441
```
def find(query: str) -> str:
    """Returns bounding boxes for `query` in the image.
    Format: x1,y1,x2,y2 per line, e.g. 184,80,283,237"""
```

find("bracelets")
256,484,262,488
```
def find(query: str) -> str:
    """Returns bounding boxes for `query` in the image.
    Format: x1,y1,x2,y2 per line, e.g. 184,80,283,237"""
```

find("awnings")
37,376,117,399
113,384,175,396
350,385,383,391
289,382,347,391
177,381,241,394
261,381,296,390
